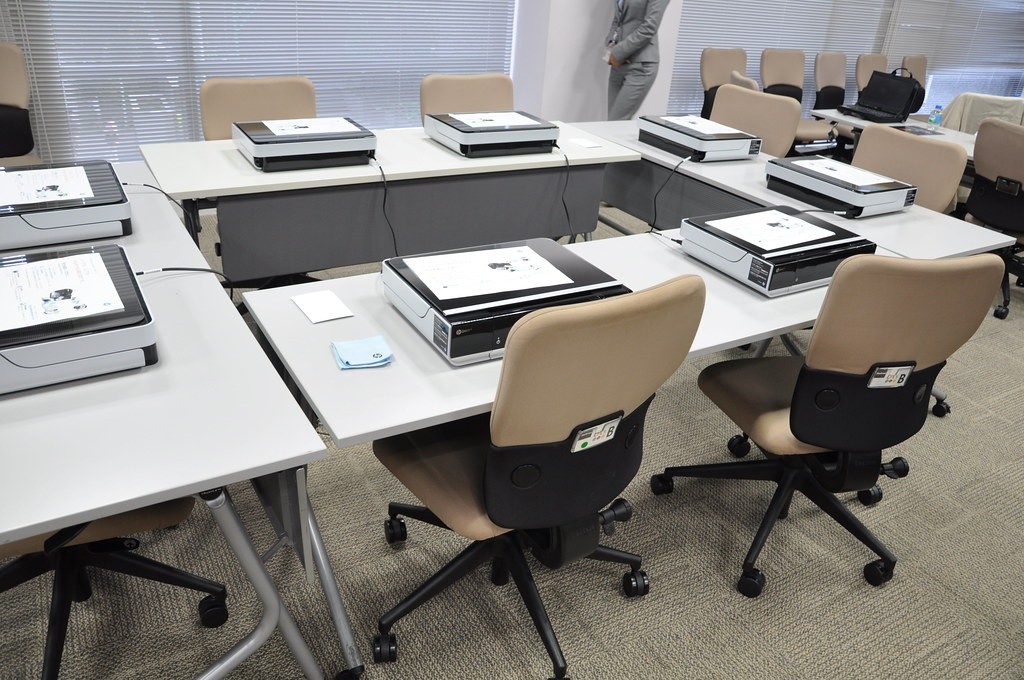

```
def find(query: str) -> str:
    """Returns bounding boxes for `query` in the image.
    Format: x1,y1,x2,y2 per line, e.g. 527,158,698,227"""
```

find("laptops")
838,71,917,118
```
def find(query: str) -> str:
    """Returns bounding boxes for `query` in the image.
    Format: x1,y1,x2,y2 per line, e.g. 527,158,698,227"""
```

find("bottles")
927,105,943,132
1015,93,1020,97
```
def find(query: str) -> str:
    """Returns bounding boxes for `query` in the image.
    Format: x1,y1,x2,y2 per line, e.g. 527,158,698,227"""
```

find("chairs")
1,497,228,675
650,253,1005,598
373,273,707,680
201,77,322,316
421,74,514,126
0,43,43,166
700,48,1024,319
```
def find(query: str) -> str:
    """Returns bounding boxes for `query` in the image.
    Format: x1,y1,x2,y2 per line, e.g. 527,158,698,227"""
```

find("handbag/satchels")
858,68,925,113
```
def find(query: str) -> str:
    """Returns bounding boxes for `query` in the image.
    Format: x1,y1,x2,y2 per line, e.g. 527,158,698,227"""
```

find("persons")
604,0,670,121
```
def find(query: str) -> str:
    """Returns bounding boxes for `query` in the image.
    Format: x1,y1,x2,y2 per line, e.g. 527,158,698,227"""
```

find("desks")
1,115,1018,680
811,109,976,169
